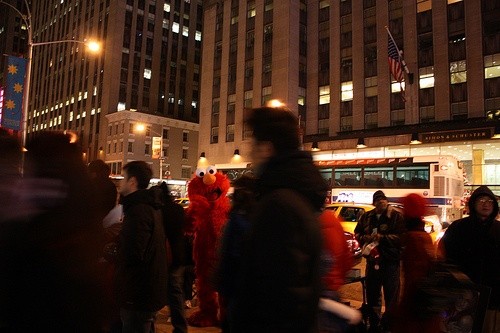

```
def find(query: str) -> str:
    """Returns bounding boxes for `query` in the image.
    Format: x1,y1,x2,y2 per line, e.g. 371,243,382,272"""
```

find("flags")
388,34,403,82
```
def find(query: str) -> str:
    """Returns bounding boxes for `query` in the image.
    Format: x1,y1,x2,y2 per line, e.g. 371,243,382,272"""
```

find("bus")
109,177,187,198
217,155,465,224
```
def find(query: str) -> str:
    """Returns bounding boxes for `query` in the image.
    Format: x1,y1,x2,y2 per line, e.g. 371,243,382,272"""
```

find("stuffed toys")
187,167,233,324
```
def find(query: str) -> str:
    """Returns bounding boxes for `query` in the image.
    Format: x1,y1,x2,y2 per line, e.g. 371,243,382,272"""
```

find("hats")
373,190,387,202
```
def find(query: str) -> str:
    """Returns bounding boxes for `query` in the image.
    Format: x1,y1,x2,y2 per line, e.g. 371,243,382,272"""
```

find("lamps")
99,146,103,151
233,149,239,155
199,152,205,157
356,140,367,149
311,141,319,149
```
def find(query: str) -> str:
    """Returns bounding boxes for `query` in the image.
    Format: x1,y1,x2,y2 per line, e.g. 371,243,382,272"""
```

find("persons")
112,161,169,333
320,208,353,300
435,186,500,333
393,194,436,333
216,107,325,333
0,128,192,333
353,190,403,333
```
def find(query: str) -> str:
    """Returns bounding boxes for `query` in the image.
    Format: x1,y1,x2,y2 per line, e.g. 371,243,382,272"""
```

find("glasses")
476,199,494,205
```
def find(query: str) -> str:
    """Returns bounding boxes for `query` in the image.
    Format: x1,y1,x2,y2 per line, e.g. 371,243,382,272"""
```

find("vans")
327,202,375,278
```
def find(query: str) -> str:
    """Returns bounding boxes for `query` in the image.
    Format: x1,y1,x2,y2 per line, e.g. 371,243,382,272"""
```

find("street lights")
19,28,102,177
133,121,163,186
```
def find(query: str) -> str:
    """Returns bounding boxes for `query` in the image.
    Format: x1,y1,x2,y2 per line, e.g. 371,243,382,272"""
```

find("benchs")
328,176,428,188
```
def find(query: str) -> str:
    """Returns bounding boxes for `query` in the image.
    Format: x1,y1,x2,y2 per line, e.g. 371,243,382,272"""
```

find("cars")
175,198,189,205
